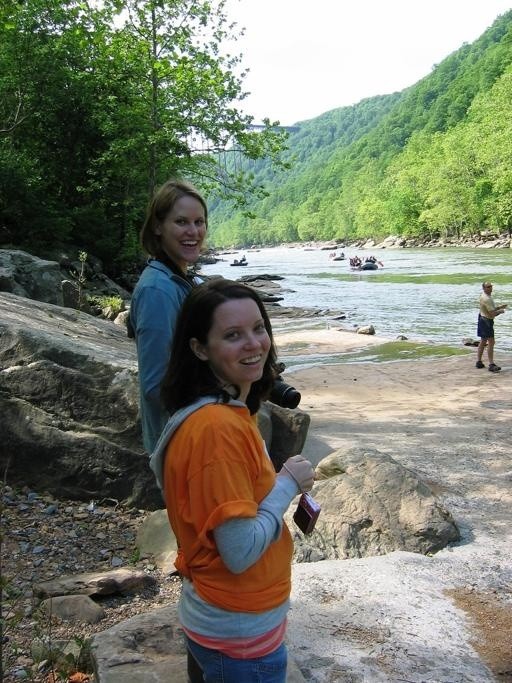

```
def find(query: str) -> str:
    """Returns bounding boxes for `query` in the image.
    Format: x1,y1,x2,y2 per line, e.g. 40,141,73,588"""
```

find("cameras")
260,362,301,410
292,493,321,535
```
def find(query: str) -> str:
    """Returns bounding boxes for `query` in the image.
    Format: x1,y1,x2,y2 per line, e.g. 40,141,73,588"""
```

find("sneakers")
488,362,502,371
476,361,485,368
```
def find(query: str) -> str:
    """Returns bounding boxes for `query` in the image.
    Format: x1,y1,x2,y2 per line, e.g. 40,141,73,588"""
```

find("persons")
330,251,344,257
476,281,507,371
350,254,376,266
145,277,318,683
127,178,208,457
240,255,246,263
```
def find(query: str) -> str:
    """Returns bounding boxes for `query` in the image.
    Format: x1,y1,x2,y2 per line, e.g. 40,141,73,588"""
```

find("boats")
230,262,248,266
334,256,343,261
361,262,378,270
